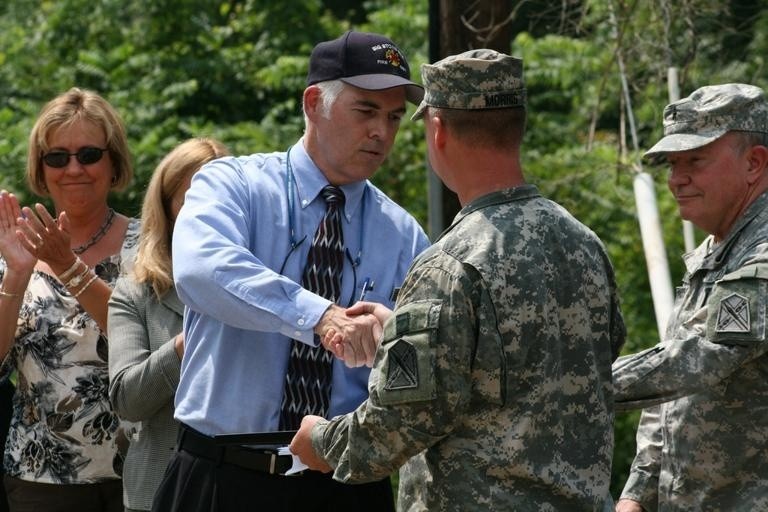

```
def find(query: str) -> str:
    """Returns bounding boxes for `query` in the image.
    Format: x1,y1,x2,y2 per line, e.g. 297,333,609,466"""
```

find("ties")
279,185,346,432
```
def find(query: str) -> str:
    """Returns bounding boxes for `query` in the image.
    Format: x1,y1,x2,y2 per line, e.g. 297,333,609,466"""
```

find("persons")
288,50,626,511
611,83,768,512
0,88,142,511
148,31,431,511
106,136,231,512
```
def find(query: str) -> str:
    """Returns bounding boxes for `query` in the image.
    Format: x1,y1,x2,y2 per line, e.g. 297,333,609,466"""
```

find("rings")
35,240,45,249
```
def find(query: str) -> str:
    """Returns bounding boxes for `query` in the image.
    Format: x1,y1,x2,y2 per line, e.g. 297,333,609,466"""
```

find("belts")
177,425,334,479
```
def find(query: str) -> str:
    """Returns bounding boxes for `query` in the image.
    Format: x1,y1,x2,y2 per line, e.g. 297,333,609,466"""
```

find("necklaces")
66,205,117,255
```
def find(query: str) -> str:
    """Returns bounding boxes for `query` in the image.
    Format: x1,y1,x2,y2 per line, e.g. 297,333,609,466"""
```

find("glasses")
41,147,111,168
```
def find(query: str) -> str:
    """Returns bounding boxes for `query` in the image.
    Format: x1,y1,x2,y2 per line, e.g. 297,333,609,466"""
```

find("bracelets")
63,264,90,287
58,257,81,280
1,290,23,299
73,275,102,296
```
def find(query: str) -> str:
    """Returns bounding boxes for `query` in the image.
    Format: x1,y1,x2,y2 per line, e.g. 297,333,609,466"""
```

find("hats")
640,83,768,167
410,49,526,121
307,31,424,105
